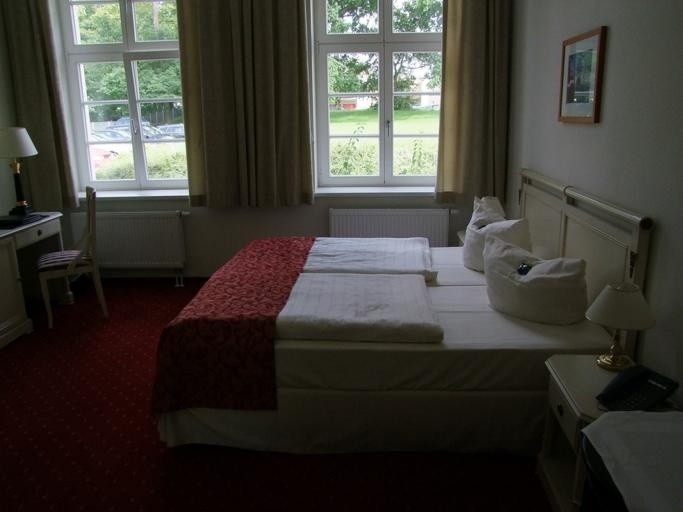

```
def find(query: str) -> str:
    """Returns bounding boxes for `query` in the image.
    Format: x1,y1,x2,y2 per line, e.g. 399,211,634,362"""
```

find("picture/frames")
557,24,609,124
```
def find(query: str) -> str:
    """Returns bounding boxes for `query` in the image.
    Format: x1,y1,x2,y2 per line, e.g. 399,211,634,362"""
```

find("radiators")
62,209,191,288
328,207,460,247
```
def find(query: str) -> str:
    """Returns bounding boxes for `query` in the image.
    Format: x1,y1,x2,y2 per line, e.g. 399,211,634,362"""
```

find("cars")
90,116,184,141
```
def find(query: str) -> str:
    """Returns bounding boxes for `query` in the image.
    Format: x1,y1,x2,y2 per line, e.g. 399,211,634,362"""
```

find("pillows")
482,235,588,325
462,196,533,273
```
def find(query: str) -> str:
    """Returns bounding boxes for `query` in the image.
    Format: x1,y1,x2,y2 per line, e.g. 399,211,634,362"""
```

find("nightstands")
456,230,467,246
536,352,676,511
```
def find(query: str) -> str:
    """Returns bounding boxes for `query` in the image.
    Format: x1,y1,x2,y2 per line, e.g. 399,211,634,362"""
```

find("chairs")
36,185,111,330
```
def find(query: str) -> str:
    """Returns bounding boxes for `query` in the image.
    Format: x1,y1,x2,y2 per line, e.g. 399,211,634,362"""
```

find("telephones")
596,364,680,411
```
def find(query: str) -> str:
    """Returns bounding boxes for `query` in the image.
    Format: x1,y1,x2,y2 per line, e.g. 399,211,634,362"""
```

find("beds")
153,167,655,455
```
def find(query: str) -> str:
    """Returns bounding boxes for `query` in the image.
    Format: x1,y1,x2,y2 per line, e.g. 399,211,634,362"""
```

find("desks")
0,211,75,351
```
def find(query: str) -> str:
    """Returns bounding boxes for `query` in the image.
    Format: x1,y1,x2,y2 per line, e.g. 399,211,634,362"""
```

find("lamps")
0,126,50,230
584,281,656,372
